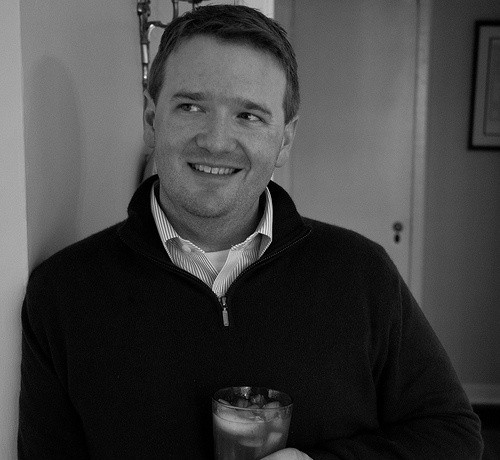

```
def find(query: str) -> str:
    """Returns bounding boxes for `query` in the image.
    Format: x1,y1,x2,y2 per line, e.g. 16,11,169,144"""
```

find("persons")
17,5,483,460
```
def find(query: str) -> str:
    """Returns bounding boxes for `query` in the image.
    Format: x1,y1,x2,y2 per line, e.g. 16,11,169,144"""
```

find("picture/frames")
467,20,500,151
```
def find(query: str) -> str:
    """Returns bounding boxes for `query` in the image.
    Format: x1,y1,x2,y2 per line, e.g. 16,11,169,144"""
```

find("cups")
211,386,293,460
